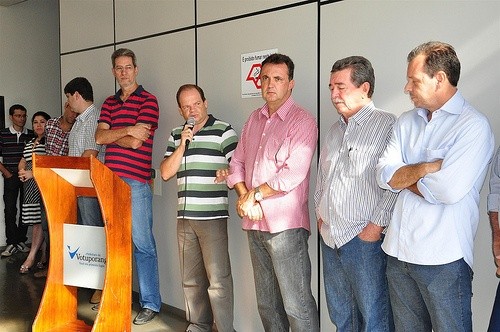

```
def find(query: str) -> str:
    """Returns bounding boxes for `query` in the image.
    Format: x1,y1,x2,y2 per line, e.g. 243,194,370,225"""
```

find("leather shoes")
134,308,157,324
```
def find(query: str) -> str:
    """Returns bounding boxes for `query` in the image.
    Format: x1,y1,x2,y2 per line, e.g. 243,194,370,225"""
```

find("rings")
23,177,26,180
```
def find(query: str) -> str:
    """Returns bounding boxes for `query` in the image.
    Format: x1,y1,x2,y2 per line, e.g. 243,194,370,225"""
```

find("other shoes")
20,262,35,273
90,289,103,303
37,261,47,268
92,304,100,311
34,271,48,278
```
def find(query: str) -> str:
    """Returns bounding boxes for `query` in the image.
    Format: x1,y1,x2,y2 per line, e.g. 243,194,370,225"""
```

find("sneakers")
17,241,32,252
1,244,16,256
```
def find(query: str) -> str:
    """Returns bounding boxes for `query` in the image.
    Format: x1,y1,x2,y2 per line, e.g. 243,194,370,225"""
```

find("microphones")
185,116,196,151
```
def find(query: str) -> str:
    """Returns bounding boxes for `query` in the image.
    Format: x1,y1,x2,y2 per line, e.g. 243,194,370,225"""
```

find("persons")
375,41,495,332
228,54,320,332
314,56,400,332
44,101,80,156
95,48,160,326
17,111,51,274
64,76,105,304
160,83,239,332
484,146,500,332
0,104,36,257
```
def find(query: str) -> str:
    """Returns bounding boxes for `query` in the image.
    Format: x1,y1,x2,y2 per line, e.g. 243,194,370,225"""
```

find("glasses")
12,114,28,119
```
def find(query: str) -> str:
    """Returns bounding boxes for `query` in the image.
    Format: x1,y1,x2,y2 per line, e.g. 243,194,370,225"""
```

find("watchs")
255,187,263,202
